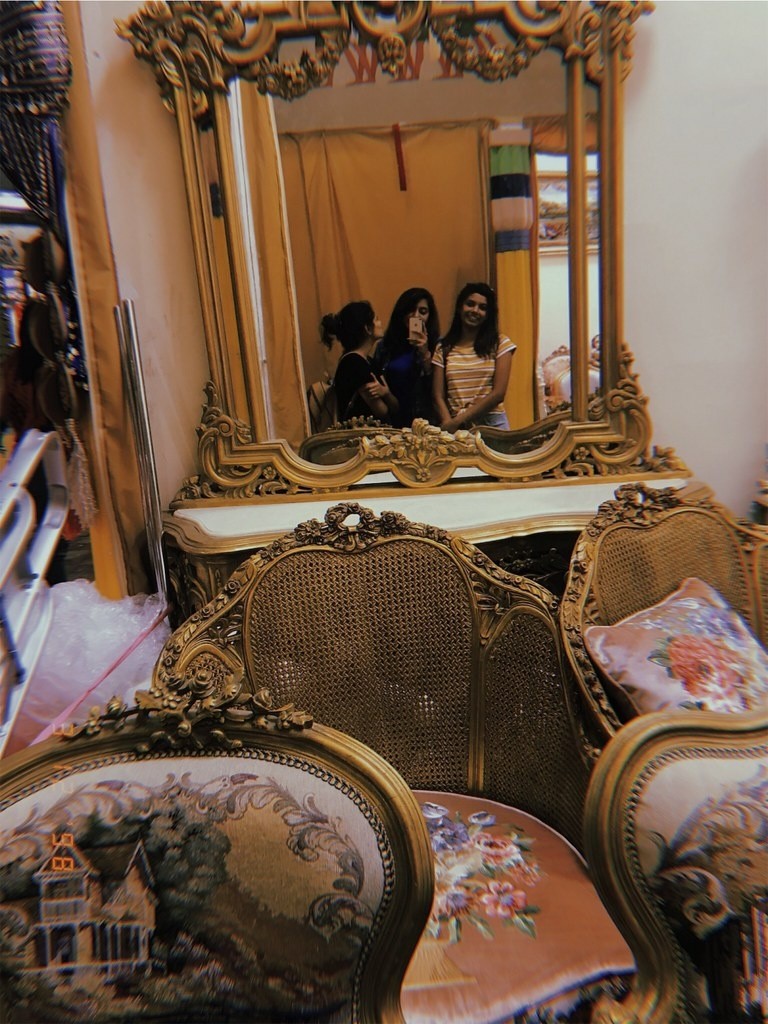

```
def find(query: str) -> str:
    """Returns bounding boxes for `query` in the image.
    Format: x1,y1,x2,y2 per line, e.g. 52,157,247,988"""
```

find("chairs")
151,502,625,859
580,709,768,1024
0,637,437,1024
557,483,768,748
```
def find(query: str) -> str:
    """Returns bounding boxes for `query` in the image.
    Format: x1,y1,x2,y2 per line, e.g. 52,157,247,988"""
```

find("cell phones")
409,317,423,345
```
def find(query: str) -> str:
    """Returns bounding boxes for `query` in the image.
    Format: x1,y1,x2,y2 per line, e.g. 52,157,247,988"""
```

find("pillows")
583,577,768,718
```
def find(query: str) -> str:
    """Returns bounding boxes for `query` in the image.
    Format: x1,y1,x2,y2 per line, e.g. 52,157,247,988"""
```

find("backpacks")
309,352,369,435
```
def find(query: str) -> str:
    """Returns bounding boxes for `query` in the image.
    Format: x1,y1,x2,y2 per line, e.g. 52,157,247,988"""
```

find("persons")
374,287,446,429
431,282,517,432
318,299,401,422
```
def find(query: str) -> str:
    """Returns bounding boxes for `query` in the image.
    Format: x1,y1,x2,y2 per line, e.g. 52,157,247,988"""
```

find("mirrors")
112,1,692,511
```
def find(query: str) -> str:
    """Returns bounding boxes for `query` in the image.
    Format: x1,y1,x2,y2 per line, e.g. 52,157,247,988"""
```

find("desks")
399,784,633,1024
160,477,687,632
